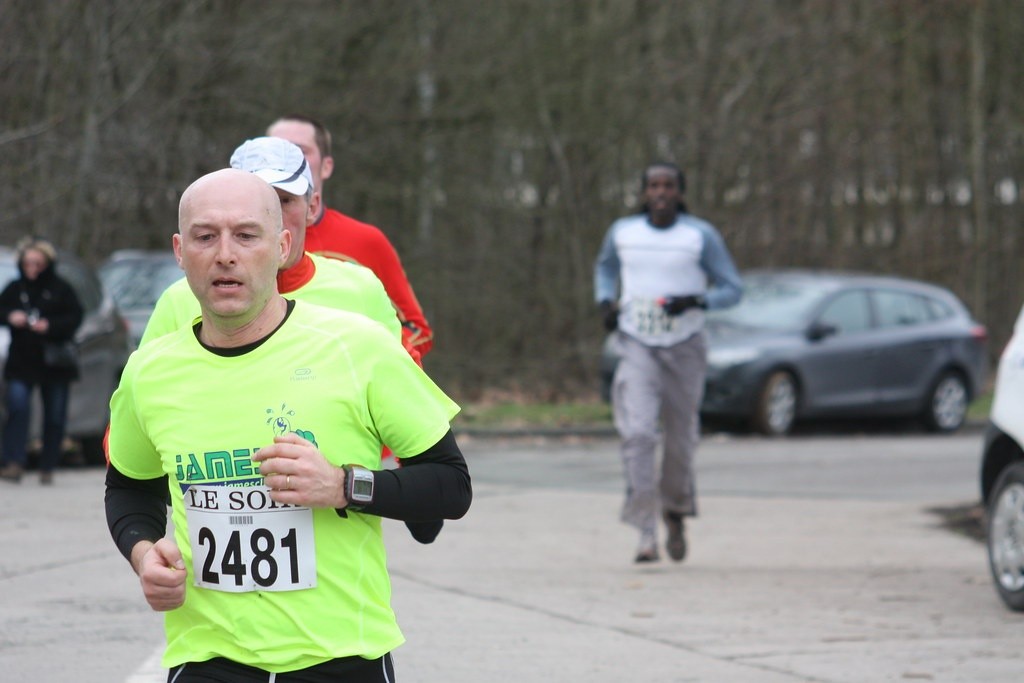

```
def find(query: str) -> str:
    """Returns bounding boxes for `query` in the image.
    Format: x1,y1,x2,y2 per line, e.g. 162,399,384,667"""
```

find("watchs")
341,463,375,513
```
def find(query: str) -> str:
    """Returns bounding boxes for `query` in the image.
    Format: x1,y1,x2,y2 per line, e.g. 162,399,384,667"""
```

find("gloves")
661,294,696,316
600,301,619,332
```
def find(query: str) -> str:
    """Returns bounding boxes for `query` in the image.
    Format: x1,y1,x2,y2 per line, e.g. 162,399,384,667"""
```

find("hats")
229,136,314,197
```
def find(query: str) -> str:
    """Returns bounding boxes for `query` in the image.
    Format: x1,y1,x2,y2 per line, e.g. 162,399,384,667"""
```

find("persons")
589,161,745,564
138,135,402,371
102,168,472,683
0,238,88,485
260,113,436,470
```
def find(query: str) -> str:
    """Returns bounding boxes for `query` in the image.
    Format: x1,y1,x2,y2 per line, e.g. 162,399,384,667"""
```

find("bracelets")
285,474,291,491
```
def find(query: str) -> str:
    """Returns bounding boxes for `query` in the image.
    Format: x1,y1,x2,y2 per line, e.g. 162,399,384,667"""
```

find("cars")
596,264,988,440
979,298,1024,612
96,247,188,346
0,246,136,472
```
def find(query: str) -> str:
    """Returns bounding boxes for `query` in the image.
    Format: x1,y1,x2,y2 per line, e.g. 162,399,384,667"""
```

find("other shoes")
662,511,685,560
2,462,23,480
40,472,50,483
635,541,657,561
